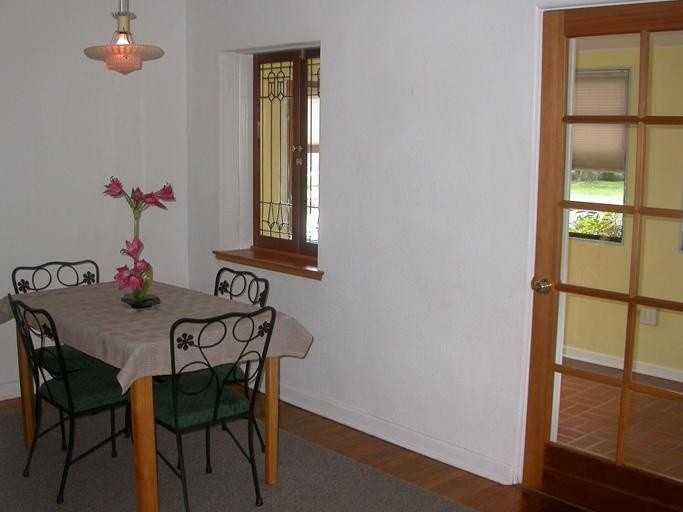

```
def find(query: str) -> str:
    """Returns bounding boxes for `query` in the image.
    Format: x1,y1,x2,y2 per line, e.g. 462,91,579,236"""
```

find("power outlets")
639,306,658,326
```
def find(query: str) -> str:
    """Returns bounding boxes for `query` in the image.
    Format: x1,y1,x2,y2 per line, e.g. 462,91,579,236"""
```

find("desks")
9,280,314,512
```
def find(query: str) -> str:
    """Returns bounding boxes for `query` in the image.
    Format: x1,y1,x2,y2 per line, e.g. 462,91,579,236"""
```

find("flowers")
103,175,176,293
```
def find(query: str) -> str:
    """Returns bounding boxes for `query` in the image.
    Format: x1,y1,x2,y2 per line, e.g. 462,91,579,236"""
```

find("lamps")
84,0,164,75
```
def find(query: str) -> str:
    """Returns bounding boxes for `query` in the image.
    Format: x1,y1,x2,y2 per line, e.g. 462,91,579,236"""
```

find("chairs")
152,266,271,469
153,306,276,512
7,293,129,504
11,259,131,450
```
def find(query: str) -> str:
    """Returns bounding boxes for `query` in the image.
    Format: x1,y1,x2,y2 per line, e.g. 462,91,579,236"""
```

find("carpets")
1,404,479,512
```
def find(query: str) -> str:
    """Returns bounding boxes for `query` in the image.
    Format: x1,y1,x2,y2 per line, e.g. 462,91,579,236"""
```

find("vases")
132,264,153,297
